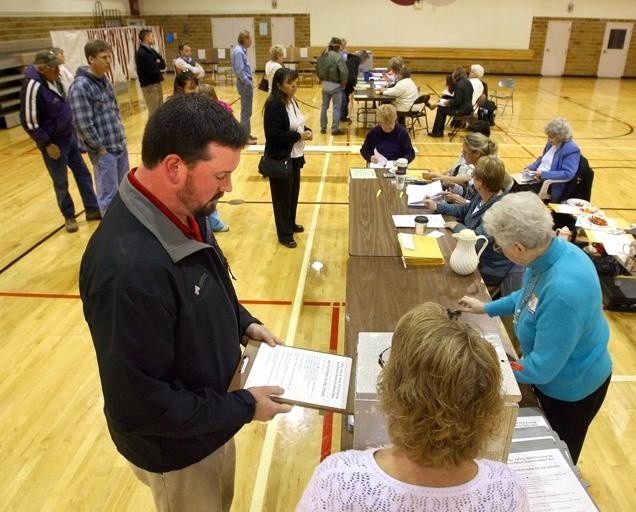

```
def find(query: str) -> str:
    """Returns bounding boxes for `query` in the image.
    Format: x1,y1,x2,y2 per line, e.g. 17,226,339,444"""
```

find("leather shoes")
285,240,297,248
296,225,304,232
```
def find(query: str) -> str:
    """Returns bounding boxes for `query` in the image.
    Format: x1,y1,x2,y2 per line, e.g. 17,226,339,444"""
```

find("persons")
260,67,313,249
453,190,613,467
172,41,205,80
76,90,294,512
361,104,416,169
231,30,258,145
294,298,532,511
197,83,234,115
173,68,230,233
424,64,484,137
316,37,369,136
379,56,419,129
422,120,515,287
18,38,130,233
135,29,166,117
265,44,285,95
509,116,580,204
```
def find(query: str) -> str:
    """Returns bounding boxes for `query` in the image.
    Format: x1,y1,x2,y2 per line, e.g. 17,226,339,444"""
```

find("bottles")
364,72,370,81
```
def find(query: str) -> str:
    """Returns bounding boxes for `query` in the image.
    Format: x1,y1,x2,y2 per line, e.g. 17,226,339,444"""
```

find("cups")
393,158,407,178
394,169,404,192
369,79,374,88
369,71,372,78
414,216,428,236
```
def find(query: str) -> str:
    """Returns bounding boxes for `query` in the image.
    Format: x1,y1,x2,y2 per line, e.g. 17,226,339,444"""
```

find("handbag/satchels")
258,156,294,180
257,77,270,92
478,101,497,125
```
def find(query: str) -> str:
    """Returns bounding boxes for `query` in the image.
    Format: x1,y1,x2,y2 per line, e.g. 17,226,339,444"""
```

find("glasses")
493,243,505,253
378,345,392,368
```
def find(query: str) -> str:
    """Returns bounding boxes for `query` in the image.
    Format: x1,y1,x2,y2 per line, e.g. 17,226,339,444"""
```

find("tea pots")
447,227,488,278
624,241,636,261
554,225,571,241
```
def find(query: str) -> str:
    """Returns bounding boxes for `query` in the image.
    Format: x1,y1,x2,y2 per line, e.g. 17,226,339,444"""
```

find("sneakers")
246,135,257,144
65,217,78,232
332,131,344,135
321,130,326,134
220,226,229,232
85,212,101,220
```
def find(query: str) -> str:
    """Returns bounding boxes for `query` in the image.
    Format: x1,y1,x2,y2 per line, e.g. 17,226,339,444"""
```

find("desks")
348,168,473,256
343,254,539,406
546,198,600,245
351,398,599,512
354,68,396,135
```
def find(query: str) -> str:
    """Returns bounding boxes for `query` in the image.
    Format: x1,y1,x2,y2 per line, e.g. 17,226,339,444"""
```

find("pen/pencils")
415,182,428,185
400,191,404,198
376,188,381,198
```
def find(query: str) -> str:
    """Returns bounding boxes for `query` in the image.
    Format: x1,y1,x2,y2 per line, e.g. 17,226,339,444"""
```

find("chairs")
489,78,515,115
445,94,486,142
551,156,594,203
396,95,430,139
296,62,315,88
217,56,237,86
557,211,576,242
488,264,517,300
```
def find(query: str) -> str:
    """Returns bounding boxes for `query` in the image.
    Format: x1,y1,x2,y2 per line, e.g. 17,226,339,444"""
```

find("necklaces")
511,272,541,324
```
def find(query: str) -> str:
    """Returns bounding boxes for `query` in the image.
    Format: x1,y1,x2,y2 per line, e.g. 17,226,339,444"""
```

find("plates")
586,216,610,229
567,197,590,210
387,168,408,174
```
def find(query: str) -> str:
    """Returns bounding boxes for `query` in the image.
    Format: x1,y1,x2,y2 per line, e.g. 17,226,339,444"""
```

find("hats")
329,38,342,48
33,51,64,66
